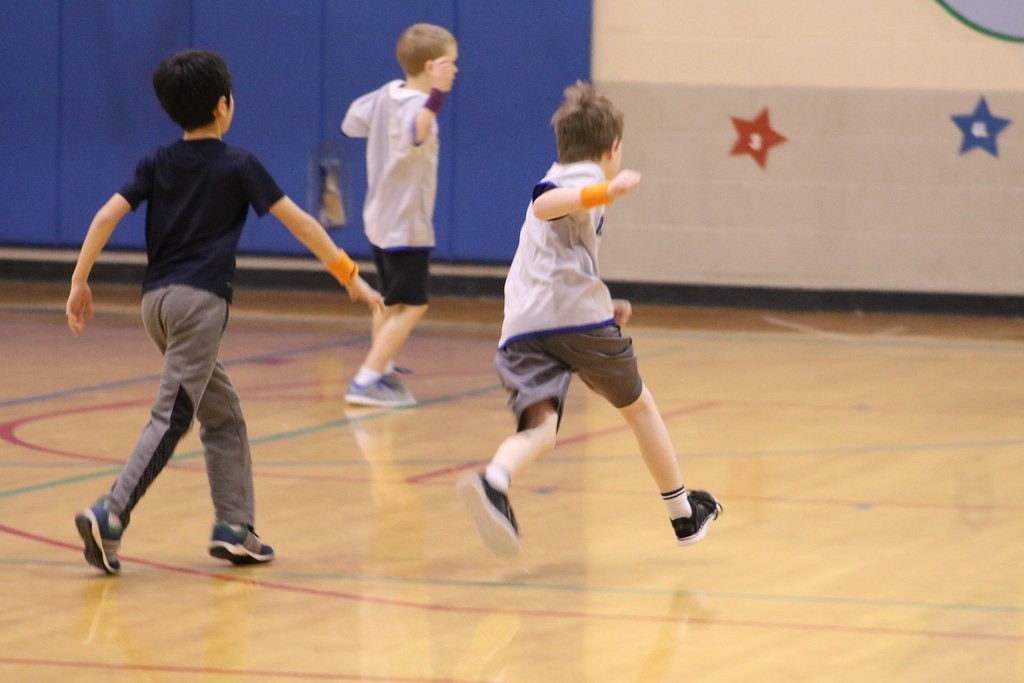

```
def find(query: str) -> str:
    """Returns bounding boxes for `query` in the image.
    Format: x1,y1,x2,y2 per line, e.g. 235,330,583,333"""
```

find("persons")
66,51,384,575
341,22,458,408
457,80,722,560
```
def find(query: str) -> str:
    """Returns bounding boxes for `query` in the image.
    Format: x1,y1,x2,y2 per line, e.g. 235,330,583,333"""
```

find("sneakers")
75,505,124,574
456,470,521,558
208,519,275,564
669,488,723,548
344,366,418,407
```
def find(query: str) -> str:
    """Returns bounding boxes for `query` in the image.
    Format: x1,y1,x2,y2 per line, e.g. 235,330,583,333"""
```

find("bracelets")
323,248,358,285
424,88,444,113
581,181,613,209
